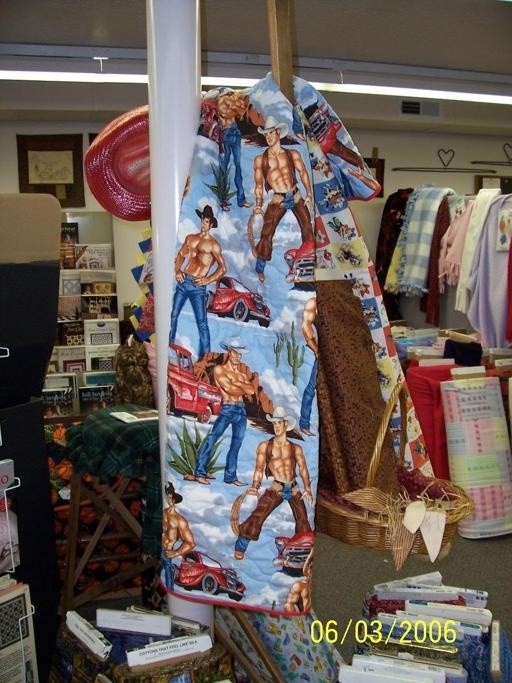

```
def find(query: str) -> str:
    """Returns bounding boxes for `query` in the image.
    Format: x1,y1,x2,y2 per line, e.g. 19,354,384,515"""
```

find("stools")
61,407,161,620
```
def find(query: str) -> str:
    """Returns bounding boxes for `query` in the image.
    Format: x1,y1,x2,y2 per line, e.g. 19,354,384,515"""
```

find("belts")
222,400,246,409
272,479,298,488
274,186,299,197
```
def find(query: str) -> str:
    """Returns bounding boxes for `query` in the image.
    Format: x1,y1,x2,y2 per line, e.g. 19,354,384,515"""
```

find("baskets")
315,373,473,556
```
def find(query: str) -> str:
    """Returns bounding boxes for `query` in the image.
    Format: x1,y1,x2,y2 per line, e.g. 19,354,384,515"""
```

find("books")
58,222,117,318
39,318,123,419
339,570,512,682
50,603,214,683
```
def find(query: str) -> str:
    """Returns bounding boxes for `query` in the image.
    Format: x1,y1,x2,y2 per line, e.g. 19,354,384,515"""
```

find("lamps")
0,42,512,105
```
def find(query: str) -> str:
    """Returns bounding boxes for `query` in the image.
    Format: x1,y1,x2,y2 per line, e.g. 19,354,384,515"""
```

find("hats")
257,116,289,139
196,203,218,227
220,335,250,354
164,481,183,503
265,406,296,432
219,86,237,96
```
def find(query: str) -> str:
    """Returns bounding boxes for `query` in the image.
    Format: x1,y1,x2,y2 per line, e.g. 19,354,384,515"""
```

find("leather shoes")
196,477,211,485
258,272,265,282
235,550,245,560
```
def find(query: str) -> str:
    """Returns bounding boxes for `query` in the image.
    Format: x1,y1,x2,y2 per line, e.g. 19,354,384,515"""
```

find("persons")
330,274,427,472
